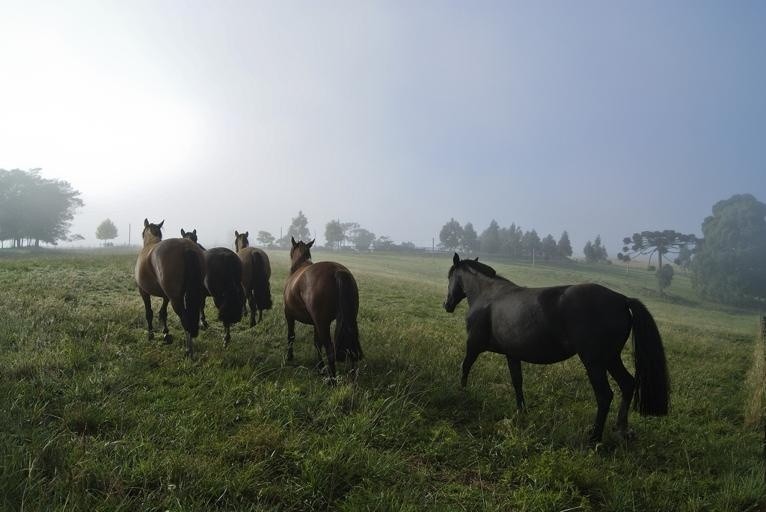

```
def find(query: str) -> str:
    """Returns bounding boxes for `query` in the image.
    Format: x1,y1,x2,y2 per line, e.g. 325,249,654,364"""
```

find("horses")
134,217,209,350
283,235,364,389
231,230,272,329
443,251,671,452
179,228,245,349
